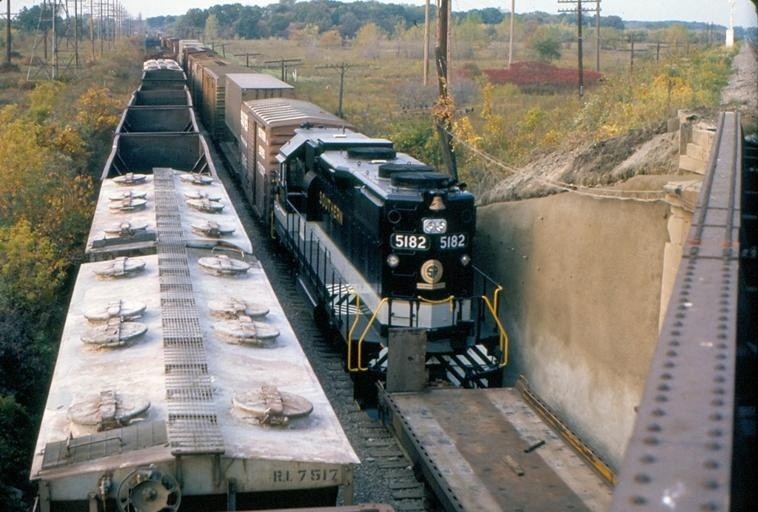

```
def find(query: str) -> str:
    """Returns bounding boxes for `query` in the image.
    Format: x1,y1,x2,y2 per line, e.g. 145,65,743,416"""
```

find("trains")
169,33,509,412
27,54,365,512
